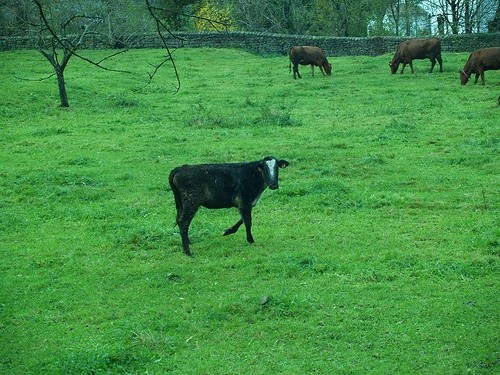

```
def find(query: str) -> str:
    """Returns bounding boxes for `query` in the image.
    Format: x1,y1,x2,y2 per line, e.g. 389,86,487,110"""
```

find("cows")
459,47,500,86
168,155,289,257
389,37,443,74
288,46,332,79
